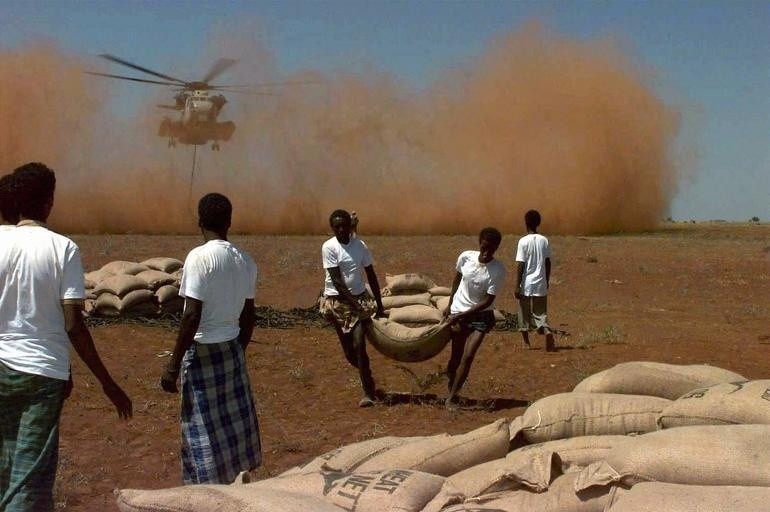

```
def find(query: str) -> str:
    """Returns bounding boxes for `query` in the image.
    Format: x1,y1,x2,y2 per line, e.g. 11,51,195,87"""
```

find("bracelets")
165,365,180,373
103,377,113,389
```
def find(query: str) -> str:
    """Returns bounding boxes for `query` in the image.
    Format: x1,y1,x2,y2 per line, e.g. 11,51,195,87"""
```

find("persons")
443,226,506,412
514,210,555,352
349,210,358,239
319,208,383,408
158,192,264,485
0,174,22,225
1,161,134,512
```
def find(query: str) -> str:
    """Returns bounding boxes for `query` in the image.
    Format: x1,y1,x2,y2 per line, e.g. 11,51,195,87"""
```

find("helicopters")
81,53,328,151
